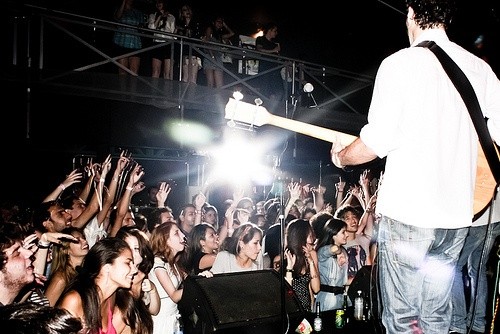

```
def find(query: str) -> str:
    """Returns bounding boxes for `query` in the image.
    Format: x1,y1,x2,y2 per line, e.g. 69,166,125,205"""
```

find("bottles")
354,290,363,320
363,292,371,322
313,302,322,332
342,294,350,324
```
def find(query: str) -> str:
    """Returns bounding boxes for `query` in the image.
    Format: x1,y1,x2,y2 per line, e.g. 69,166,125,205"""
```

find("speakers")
347,264,373,321
178,269,306,334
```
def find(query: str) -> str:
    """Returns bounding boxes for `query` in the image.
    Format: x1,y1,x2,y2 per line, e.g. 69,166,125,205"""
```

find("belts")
320,284,346,295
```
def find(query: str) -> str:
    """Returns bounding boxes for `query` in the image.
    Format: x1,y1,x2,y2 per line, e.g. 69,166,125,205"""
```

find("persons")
0,150,500,334
337,0,500,334
113,0,302,103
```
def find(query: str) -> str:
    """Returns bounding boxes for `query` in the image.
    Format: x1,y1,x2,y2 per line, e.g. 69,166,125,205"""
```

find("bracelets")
311,273,320,279
338,191,344,192
39,245,50,249
286,269,294,272
126,186,133,191
100,178,106,183
227,229,234,233
38,240,51,246
366,207,372,212
60,183,66,190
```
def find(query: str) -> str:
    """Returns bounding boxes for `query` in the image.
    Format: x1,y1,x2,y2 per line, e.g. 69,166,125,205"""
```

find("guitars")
223,88,500,224
